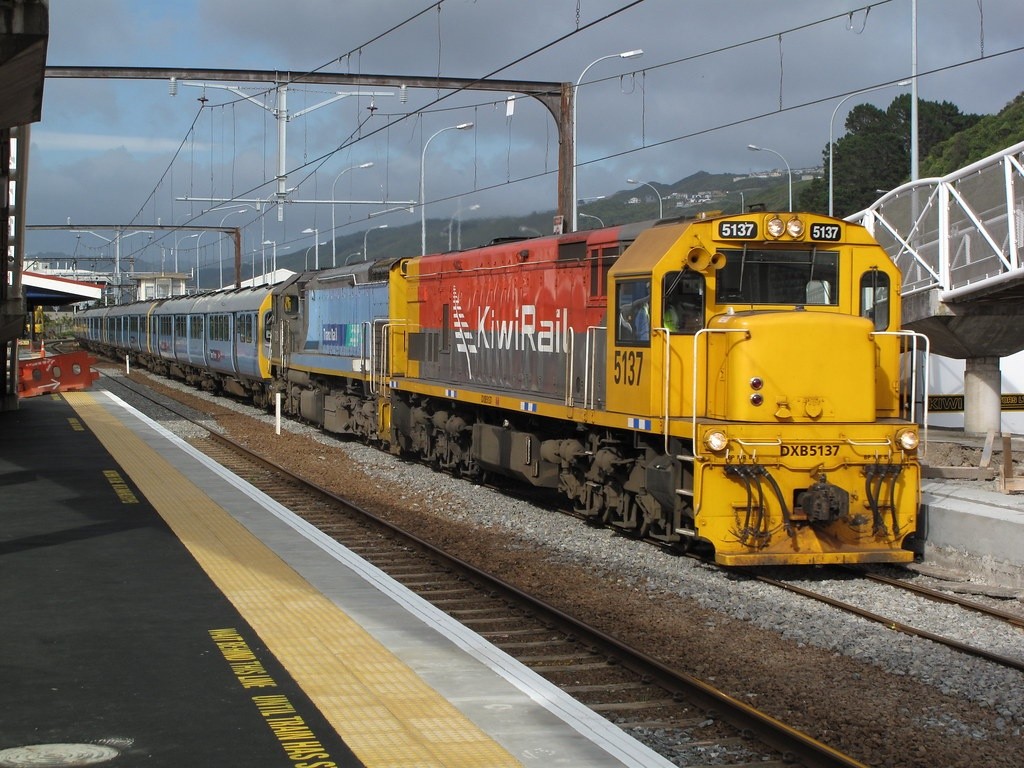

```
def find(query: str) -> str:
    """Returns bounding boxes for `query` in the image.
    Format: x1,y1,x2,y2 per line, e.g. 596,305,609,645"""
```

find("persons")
636,282,679,340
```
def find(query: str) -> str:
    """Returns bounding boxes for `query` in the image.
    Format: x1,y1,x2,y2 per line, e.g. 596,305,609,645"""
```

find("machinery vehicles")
24,305,55,340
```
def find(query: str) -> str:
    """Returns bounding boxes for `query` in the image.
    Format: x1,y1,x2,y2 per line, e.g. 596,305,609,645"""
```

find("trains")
74,213,929,570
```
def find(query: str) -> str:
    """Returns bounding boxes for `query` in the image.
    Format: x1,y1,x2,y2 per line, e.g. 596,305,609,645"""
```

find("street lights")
332,162,374,268
216,209,249,290
626,179,654,218
420,122,474,257
447,205,480,252
363,224,388,262
572,49,646,239
726,190,746,214
828,80,911,223
252,240,291,284
260,187,301,285
300,228,328,273
69,227,156,307
172,213,198,273
578,212,605,228
747,144,792,211
